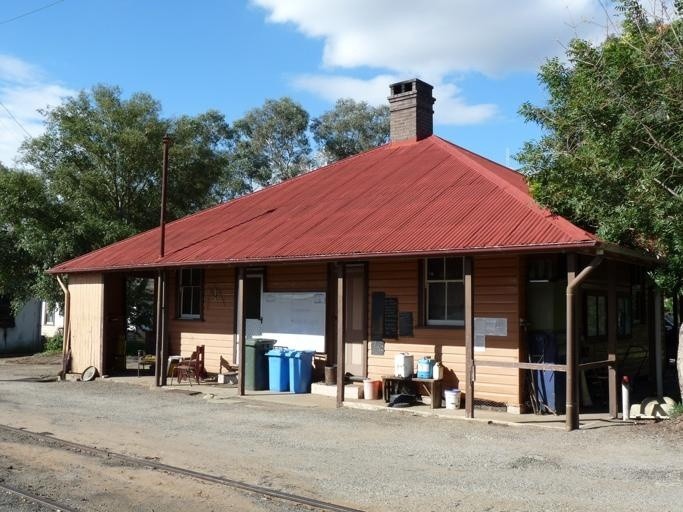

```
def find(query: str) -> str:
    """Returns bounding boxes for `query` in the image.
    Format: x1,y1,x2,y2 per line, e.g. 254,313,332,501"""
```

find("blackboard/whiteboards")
259,292,327,353
371,291,413,355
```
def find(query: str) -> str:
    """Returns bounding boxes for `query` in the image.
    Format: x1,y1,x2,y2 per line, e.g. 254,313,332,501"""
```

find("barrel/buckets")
325,364,337,385
433,361,444,380
363,379,379,399
417,356,432,379
444,388,462,409
394,352,414,378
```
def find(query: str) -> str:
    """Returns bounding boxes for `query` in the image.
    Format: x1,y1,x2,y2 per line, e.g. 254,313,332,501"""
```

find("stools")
217,372,238,384
167,356,184,378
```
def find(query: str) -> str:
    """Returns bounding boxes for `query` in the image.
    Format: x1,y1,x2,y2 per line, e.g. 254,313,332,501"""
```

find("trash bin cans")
245,338,316,393
527,329,584,413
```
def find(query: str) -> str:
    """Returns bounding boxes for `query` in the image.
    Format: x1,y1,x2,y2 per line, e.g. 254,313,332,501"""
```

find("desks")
381,374,442,410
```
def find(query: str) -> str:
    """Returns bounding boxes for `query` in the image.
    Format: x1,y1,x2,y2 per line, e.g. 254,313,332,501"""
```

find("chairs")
171,351,200,387
183,344,205,382
138,350,156,377
